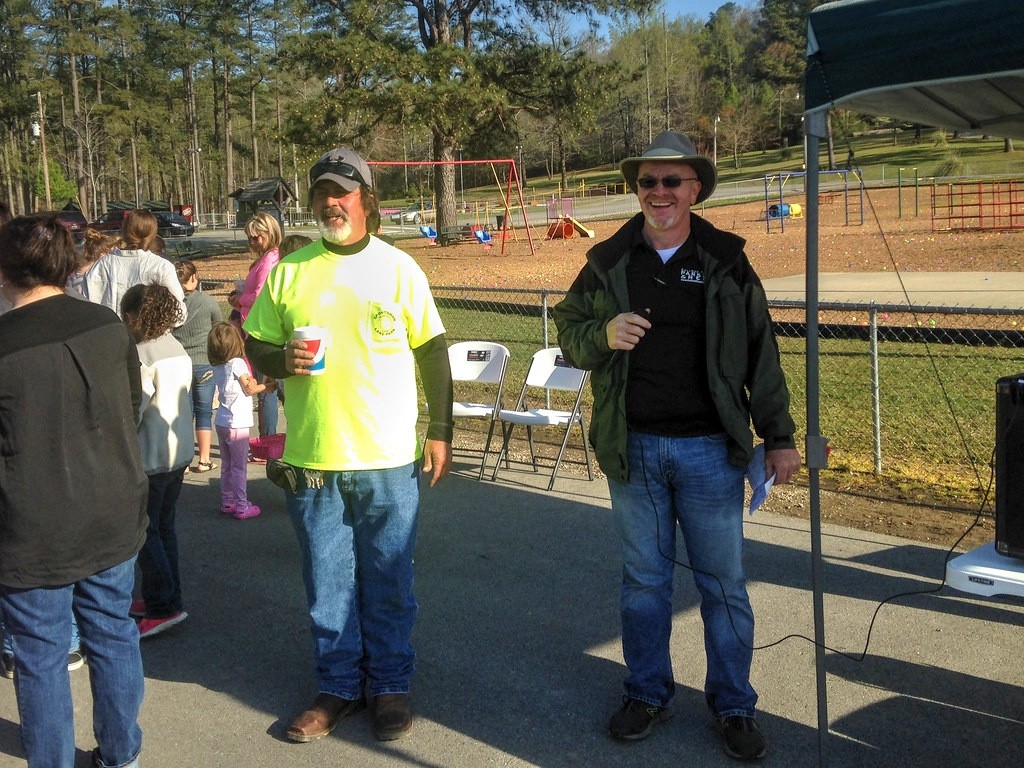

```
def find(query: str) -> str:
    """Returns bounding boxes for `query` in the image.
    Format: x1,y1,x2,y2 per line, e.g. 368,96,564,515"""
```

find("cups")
233,280,245,293
294,326,325,376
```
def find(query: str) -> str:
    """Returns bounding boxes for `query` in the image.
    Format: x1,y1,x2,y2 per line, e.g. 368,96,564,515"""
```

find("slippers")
246,447,268,464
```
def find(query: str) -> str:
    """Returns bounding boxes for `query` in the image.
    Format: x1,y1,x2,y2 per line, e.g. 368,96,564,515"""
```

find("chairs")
490,347,594,492
420,226,437,246
474,230,492,250
421,340,511,480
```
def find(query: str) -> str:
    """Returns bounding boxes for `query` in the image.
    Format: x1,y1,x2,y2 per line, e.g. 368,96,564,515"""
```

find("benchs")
440,224,501,248
174,240,203,262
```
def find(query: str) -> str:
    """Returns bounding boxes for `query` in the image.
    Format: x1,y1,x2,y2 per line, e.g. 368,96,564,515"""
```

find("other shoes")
0,654,15,679
66,647,88,671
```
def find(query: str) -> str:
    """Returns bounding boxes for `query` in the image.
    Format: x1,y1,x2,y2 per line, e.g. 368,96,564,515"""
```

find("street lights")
714,117,721,167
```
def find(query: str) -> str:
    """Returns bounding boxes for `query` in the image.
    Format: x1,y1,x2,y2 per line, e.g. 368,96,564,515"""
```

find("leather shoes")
370,692,412,740
288,692,366,741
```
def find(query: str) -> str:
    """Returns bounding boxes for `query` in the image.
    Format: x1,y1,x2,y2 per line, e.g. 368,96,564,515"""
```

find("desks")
947,541,1024,598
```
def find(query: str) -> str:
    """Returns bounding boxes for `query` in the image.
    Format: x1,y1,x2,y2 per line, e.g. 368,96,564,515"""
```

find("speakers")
995,372,1024,560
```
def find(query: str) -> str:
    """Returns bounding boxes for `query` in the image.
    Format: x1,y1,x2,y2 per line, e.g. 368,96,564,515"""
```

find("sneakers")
608,695,674,741
137,611,188,639
129,596,148,615
720,715,766,760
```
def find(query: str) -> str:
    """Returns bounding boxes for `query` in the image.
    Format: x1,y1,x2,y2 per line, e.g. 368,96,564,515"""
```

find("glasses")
249,233,262,241
309,161,368,188
634,175,699,189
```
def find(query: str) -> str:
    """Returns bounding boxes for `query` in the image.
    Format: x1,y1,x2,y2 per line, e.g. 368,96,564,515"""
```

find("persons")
0,228,124,677
551,129,806,760
262,233,313,402
367,210,394,247
242,147,456,744
229,212,279,437
0,215,149,767
170,260,224,475
208,324,278,517
81,208,188,329
119,279,191,639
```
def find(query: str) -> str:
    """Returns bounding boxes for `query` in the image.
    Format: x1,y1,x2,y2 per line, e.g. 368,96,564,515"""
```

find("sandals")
196,460,217,473
220,499,252,514
234,506,261,520
182,465,190,475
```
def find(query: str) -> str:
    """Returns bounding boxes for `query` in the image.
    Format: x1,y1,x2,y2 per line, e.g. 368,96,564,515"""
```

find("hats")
309,147,372,191
618,131,718,207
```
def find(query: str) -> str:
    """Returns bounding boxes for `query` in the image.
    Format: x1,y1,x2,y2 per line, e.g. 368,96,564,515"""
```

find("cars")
389,201,439,225
30,210,88,232
86,210,195,238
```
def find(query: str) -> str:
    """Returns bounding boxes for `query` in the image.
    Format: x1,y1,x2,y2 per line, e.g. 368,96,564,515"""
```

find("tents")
799,2,1023,768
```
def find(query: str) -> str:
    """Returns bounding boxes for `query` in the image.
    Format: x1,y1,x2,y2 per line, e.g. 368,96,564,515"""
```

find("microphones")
607,309,649,374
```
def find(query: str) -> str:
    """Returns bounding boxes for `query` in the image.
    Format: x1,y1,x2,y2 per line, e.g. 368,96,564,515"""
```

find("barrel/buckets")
769,204,804,218
548,222,575,239
